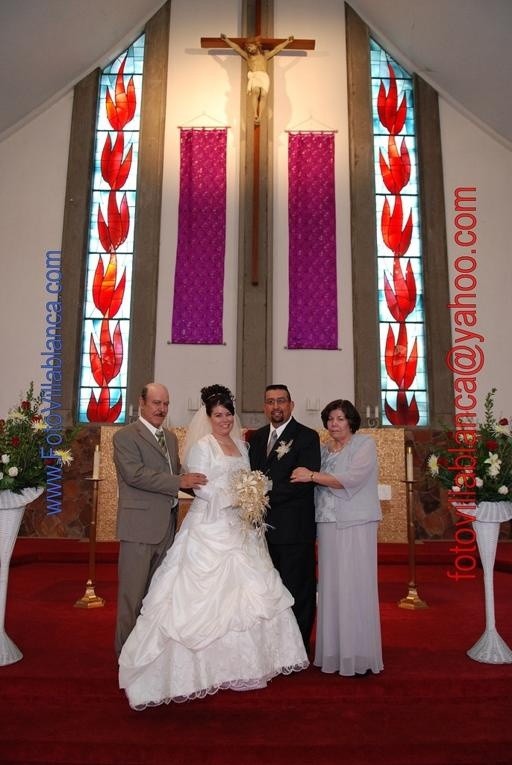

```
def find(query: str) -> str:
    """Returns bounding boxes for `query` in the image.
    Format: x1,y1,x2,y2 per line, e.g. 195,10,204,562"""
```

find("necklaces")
213,438,237,450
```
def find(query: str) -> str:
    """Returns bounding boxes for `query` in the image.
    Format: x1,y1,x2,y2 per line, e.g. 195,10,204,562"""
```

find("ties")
156,431,174,506
266,430,277,458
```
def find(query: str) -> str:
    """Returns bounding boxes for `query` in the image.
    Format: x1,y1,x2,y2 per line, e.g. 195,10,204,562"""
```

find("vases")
450,500,512,665
0,486,44,668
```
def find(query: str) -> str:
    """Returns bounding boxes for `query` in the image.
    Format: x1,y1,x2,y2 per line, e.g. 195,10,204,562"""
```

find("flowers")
0,379,81,495
423,388,512,505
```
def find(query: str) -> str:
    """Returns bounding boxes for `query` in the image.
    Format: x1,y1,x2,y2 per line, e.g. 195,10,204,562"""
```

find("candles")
92,445,101,479
188,398,201,409
129,405,142,416
406,446,414,482
307,398,320,410
366,404,380,417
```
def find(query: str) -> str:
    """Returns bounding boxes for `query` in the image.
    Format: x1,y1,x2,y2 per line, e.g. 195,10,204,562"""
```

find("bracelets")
310,470,320,483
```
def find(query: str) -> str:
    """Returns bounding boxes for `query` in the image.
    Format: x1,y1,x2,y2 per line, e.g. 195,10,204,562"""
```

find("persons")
187,384,268,687
288,398,386,679
244,382,321,662
113,382,211,684
220,32,295,125
